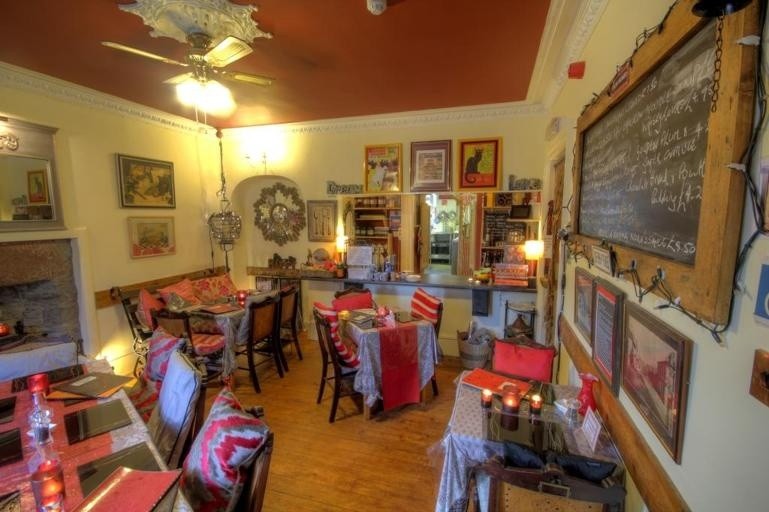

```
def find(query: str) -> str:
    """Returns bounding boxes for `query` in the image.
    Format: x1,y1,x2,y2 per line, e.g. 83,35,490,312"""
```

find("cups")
483,413,542,431
237,290,248,305
480,386,542,412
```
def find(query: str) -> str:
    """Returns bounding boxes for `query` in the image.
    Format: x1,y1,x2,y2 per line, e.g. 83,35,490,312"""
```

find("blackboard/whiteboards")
473,288,489,317
574,1,759,325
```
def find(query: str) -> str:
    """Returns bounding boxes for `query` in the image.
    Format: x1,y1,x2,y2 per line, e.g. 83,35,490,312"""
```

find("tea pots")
578,373,600,415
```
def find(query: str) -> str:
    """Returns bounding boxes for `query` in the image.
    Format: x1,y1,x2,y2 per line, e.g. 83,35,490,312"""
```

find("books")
198,302,242,315
0,363,184,512
461,363,573,459
343,306,422,329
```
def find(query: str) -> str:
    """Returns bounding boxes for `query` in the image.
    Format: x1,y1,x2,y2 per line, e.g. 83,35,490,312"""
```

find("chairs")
311,287,444,424
465,449,626,512
486,333,558,382
145,353,206,470
109,266,304,390
2,343,84,394
125,327,187,422
179,390,274,512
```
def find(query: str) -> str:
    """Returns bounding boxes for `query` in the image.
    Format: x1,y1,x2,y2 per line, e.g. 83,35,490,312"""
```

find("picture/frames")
509,204,531,220
456,135,502,194
573,262,693,464
24,169,49,205
124,214,179,260
116,154,176,209
410,138,452,194
306,199,337,243
361,140,405,193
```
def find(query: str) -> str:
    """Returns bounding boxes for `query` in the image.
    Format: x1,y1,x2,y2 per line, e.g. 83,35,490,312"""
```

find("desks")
437,367,626,512
2,360,186,512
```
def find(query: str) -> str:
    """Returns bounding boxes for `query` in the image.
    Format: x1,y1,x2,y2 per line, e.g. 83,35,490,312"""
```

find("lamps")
163,69,232,117
207,129,243,251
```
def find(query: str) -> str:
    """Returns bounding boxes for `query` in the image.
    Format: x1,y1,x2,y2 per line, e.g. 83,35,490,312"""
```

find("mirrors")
339,194,539,277
0,152,57,223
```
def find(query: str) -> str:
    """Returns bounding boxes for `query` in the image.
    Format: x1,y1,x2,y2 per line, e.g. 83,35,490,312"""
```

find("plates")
406,274,421,283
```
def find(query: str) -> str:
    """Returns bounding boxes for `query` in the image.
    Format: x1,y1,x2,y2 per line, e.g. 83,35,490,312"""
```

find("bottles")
23,372,66,511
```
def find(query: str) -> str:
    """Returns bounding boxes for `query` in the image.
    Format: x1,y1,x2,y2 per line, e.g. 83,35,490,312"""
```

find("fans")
101,25,272,117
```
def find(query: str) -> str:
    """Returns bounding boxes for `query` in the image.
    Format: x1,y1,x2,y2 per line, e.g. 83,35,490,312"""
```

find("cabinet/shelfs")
503,300,538,340
